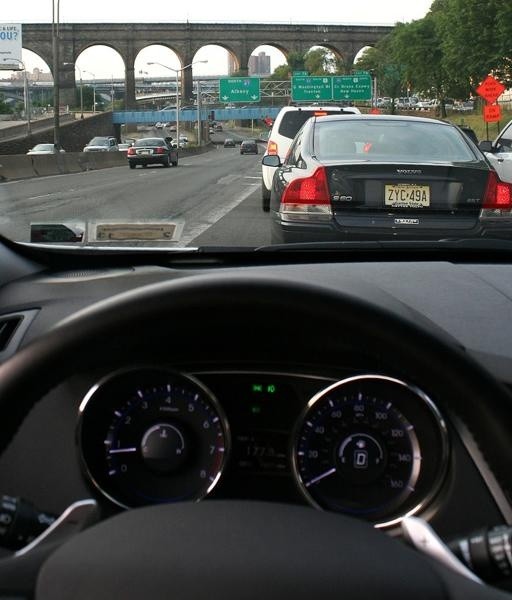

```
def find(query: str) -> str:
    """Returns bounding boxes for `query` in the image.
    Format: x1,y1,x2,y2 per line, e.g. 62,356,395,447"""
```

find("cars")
478,119,512,184
156,122,187,147
120,144,129,152
375,96,474,112
260,106,361,207
83,136,118,154
27,143,64,156
272,114,512,260
128,138,179,166
208,122,258,155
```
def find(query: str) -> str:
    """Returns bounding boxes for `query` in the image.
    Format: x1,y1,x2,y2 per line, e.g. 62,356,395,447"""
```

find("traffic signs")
333,75,372,102
220,78,259,103
292,76,332,101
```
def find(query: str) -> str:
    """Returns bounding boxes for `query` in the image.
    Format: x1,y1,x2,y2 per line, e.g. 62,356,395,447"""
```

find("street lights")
3,57,31,135
62,61,84,119
147,59,208,138
